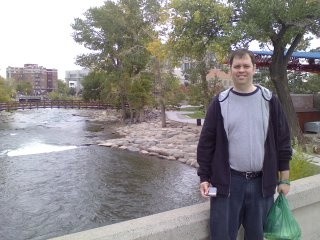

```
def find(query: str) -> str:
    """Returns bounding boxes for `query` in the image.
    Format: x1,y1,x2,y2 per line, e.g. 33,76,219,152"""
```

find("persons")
196,47,292,240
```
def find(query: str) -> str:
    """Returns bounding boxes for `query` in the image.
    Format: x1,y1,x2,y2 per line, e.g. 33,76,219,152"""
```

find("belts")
231,168,263,180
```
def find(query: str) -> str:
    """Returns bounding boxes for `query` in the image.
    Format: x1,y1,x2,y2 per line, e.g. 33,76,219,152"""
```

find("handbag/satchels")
263,189,301,240
